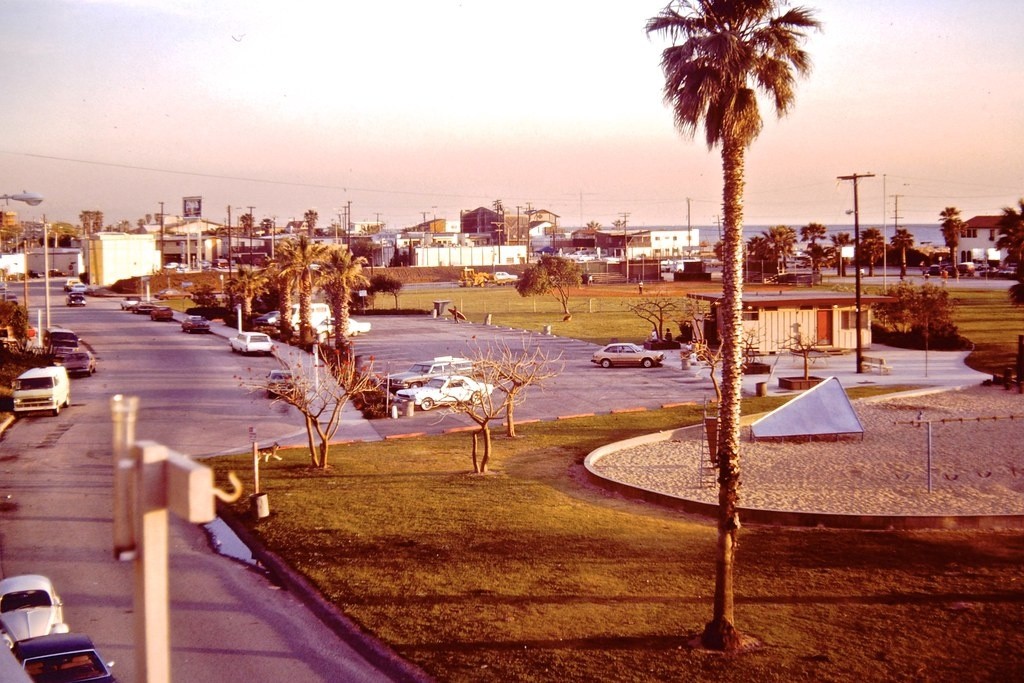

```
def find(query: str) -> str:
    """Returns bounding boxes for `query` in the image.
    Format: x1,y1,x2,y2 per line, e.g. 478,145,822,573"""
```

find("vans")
12,366,71,417
44,327,80,359
290,304,331,332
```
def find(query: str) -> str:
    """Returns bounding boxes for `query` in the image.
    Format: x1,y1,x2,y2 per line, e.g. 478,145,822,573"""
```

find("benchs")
860,355,892,375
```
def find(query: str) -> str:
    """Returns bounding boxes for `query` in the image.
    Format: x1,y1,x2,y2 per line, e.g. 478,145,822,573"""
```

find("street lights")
845,208,863,374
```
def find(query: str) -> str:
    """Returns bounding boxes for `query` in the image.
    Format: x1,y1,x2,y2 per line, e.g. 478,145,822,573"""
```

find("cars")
0,281,19,307
11,632,117,683
492,271,519,281
229,332,276,356
256,311,281,325
590,343,665,368
64,277,87,294
181,316,210,334
62,351,97,376
266,370,293,399
121,296,173,321
0,574,69,649
927,260,1018,276
385,354,494,412
316,318,370,339
66,292,86,307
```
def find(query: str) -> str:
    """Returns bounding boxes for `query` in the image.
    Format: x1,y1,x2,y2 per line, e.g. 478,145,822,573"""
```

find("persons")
638,280,644,295
664,328,674,341
452,305,459,324
650,328,658,342
588,274,592,287
